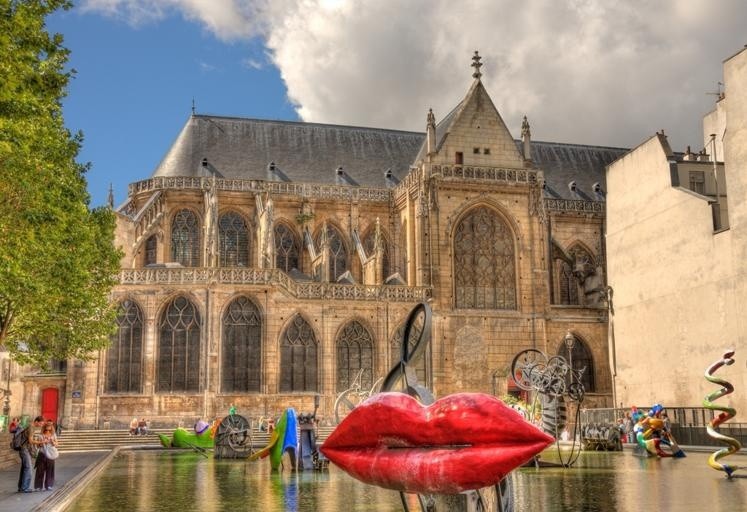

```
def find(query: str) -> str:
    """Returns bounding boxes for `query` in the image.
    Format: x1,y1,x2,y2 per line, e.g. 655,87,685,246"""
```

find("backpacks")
10,425,32,451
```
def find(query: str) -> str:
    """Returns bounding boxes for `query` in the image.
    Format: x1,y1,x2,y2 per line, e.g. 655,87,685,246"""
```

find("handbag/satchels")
43,445,60,461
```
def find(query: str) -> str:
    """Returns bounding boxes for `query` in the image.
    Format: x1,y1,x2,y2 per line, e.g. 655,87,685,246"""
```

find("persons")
9,417,18,433
258,415,266,432
130,418,138,436
33,418,59,492
625,412,636,443
264,416,275,433
138,418,147,436
15,419,23,431
17,415,48,494
617,419,627,441
